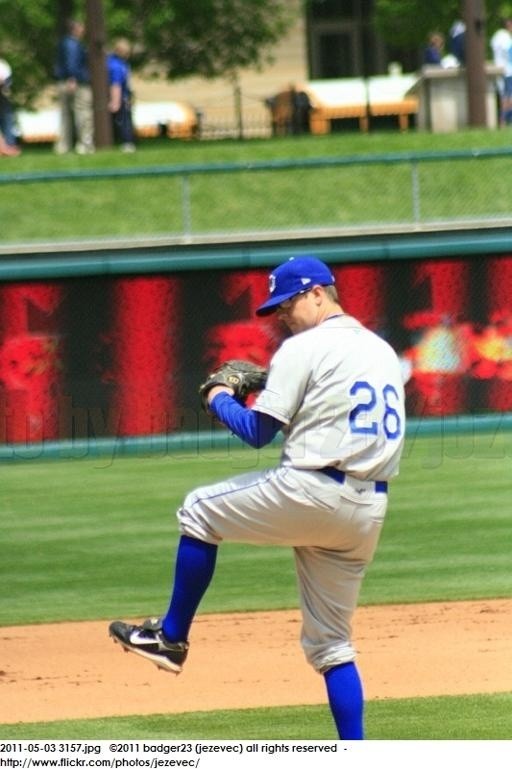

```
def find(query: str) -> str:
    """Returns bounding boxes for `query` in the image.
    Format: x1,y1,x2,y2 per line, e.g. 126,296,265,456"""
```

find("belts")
318,467,387,493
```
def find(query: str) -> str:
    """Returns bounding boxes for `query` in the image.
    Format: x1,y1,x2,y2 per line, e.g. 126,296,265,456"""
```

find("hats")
256,256,335,316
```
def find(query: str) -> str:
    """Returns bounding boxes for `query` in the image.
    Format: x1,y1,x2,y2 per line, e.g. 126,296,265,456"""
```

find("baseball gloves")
200,360,268,416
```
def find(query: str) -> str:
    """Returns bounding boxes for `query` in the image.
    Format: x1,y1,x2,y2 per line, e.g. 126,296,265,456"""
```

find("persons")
109,255,407,739
490,16,512,128
449,18,466,66
59,20,95,154
0,59,20,156
105,37,135,146
425,33,445,63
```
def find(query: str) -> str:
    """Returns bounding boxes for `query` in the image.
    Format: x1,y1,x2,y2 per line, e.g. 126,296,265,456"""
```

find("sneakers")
109,620,189,674
